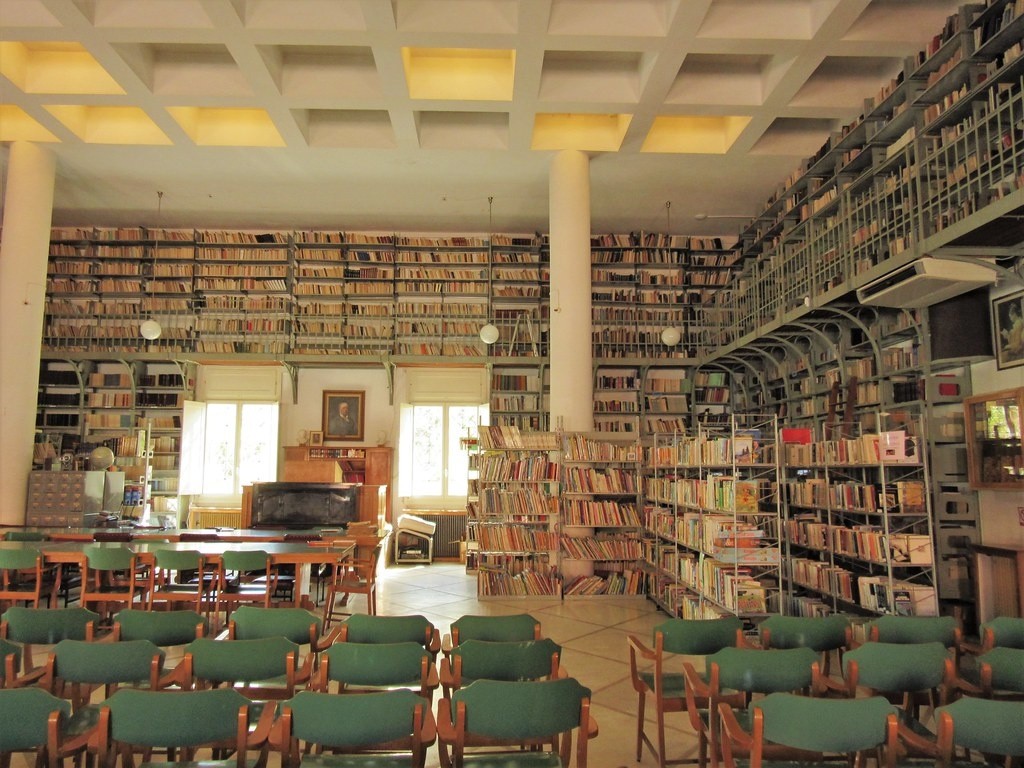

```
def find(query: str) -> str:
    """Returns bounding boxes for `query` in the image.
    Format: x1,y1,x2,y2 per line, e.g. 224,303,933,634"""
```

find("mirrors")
963,385,1024,490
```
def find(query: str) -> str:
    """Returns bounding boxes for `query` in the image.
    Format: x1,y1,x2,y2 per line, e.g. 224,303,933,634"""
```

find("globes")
90,446,115,472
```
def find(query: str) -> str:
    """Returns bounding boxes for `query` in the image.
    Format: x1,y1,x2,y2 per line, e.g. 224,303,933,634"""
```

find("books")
34,0,1024,623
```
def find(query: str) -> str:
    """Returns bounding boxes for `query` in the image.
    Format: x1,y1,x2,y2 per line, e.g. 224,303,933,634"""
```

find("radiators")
401,509,474,562
188,506,244,530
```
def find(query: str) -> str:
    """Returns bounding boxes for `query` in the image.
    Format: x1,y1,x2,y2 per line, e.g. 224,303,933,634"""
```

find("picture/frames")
309,431,324,447
321,388,365,442
991,288,1024,371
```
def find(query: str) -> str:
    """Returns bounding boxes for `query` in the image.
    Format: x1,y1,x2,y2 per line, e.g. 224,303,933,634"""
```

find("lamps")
480,197,499,345
660,201,681,348
140,190,162,341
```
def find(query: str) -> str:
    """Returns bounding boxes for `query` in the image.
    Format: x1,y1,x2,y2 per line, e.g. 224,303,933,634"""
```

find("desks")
138,540,357,615
216,527,390,567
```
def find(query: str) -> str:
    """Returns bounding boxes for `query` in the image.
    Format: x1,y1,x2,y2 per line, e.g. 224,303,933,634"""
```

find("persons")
328,401,358,435
1000,303,1024,355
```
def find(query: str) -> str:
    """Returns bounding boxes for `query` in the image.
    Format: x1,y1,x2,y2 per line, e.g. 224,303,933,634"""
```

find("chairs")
0,524,1024,768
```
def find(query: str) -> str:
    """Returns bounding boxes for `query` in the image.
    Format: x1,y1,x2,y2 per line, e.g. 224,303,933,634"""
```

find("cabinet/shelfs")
281,445,396,524
28,0,1024,626
